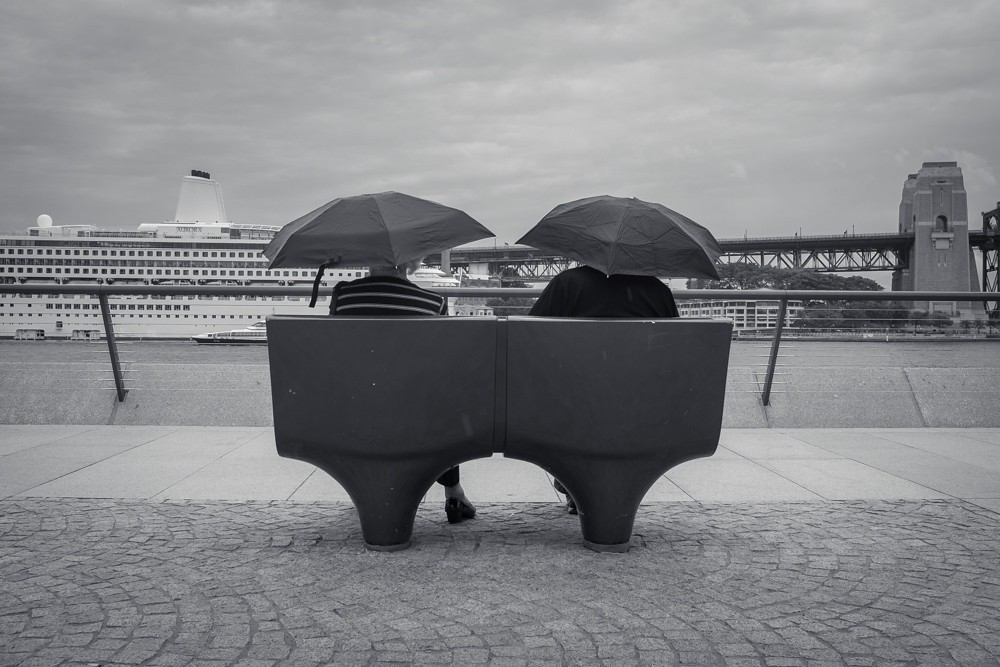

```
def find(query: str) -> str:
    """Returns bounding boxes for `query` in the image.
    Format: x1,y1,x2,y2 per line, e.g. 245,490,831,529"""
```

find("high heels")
446,498,476,524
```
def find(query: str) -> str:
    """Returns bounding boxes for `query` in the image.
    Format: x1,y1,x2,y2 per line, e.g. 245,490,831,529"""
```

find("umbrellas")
259,192,495,269
515,194,723,283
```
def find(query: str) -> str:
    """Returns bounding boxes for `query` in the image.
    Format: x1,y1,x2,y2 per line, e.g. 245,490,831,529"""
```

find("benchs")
266,313,734,552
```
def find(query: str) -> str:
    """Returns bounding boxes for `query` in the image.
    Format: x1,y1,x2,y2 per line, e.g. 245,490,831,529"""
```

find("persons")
527,266,680,514
329,255,476,523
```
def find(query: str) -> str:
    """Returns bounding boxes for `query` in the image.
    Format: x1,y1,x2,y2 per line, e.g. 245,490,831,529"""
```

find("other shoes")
566,494,578,515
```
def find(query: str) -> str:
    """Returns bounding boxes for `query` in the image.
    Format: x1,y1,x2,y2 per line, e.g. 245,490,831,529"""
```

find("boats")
190,321,268,345
0,169,495,341
